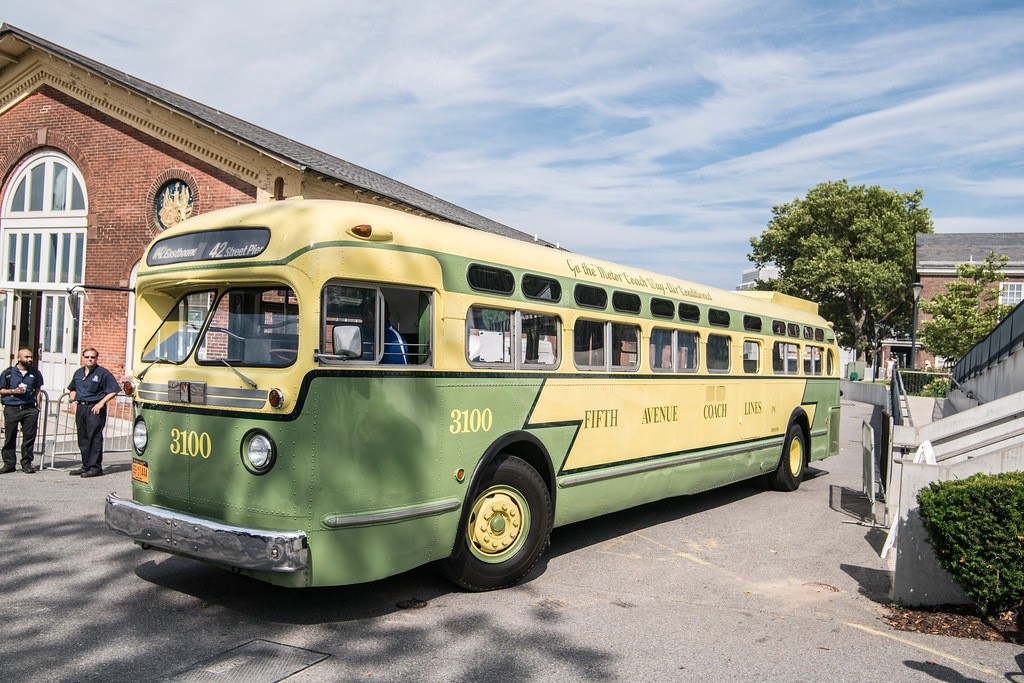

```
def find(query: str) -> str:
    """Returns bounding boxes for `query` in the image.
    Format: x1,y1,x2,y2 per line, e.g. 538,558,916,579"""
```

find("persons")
0,349,44,474
66,348,122,478
349,296,408,364
885,357,897,378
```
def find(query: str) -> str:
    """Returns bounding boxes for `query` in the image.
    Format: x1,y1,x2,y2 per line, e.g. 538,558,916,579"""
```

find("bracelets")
11,389,13,395
69,399,76,403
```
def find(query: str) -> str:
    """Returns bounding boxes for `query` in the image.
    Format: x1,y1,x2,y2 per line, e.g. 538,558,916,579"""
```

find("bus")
68,198,841,593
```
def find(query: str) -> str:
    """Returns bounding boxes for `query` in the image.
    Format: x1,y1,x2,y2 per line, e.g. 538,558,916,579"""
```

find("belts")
78,401,98,405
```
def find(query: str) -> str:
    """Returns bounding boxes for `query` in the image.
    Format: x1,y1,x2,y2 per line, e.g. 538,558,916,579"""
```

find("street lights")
872,322,881,384
911,282,924,370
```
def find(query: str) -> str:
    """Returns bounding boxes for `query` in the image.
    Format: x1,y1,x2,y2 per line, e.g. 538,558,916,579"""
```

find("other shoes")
0,466,16,474
80,470,103,477
70,468,87,475
22,462,35,473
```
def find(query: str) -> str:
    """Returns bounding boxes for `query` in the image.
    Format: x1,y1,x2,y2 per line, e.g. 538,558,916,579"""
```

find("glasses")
84,356,96,359
